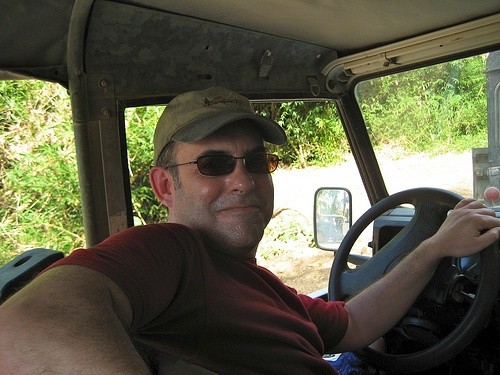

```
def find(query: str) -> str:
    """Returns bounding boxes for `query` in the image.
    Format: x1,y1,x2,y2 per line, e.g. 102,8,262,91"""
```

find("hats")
153,86,287,167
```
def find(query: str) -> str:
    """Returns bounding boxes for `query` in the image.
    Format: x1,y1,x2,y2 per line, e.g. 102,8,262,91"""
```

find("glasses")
165,154,283,177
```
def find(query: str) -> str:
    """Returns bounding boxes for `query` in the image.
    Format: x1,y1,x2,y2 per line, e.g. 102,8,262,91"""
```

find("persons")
0,87,500,375
